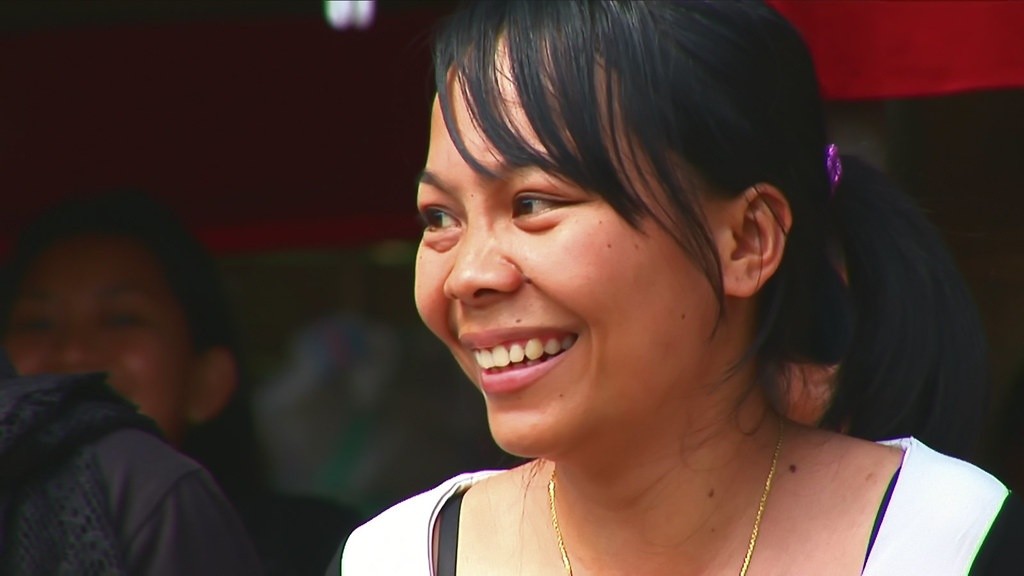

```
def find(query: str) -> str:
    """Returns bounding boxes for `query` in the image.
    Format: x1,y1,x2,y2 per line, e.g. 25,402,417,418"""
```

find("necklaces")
547,417,785,576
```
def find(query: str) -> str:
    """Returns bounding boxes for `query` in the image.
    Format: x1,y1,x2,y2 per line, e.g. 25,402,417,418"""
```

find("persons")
343,0,1024,576
0,195,363,576
